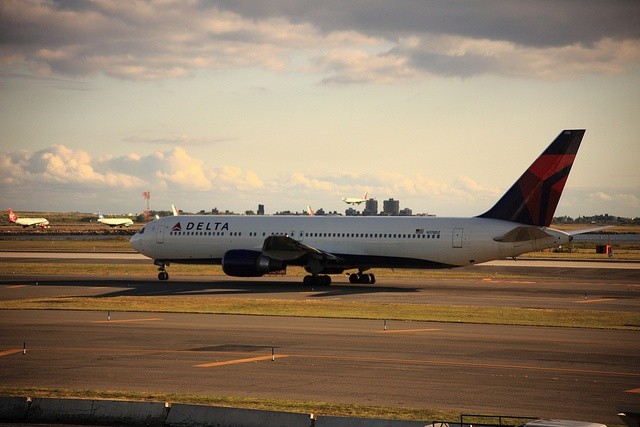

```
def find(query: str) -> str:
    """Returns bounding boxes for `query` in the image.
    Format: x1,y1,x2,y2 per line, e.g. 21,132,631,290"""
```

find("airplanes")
128,127,588,287
342,192,370,205
97,212,133,228
7,207,49,228
